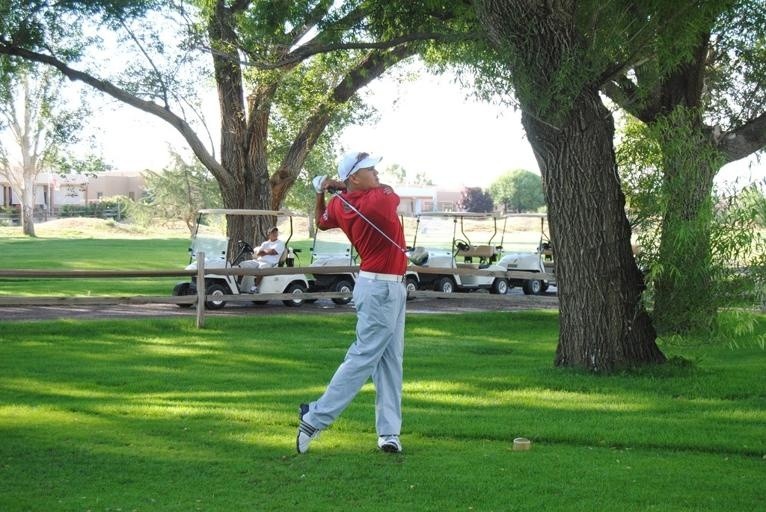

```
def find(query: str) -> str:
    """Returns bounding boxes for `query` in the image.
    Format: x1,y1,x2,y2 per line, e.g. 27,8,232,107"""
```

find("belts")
358,270,406,282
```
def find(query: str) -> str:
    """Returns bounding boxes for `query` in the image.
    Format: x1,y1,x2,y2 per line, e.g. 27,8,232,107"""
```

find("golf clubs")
327,187,428,266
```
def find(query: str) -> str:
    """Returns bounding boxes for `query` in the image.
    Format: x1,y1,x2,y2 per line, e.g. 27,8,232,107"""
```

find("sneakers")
377,432,402,454
295,401,321,454
249,285,258,294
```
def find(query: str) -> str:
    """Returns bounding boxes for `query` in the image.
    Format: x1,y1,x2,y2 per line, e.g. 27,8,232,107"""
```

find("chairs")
274,248,288,267
411,247,428,265
541,248,554,267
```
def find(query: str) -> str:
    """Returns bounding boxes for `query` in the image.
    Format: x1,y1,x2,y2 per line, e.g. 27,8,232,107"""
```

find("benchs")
455,244,493,270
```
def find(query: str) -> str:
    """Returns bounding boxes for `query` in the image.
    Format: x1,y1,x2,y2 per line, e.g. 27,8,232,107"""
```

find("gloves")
254,246,264,255
313,175,329,194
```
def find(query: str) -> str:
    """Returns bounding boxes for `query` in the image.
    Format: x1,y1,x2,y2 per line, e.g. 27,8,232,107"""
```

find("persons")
296,149,409,453
237,227,285,294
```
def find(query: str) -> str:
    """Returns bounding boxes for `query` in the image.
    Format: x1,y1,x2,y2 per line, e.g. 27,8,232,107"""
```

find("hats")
337,151,384,182
266,226,278,237
